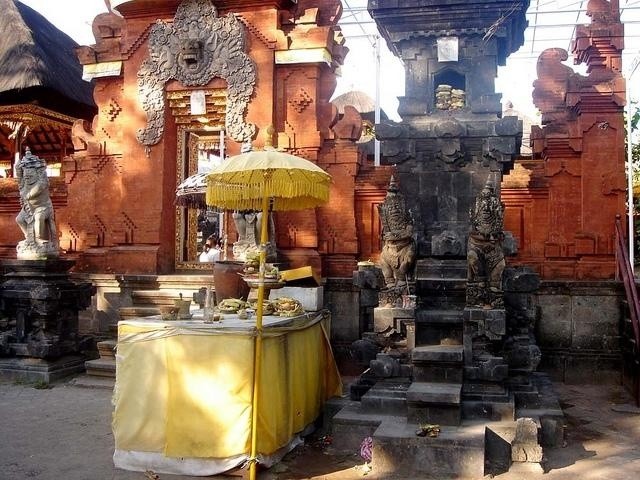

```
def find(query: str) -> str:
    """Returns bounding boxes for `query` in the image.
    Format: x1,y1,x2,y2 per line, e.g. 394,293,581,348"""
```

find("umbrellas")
204,124,334,480
174,163,272,213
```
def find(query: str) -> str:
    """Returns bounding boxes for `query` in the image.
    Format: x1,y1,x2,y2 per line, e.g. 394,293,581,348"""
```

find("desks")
111,303,333,479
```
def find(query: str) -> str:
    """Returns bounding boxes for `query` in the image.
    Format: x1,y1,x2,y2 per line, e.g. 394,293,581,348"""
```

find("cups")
245,309,255,318
159,306,179,320
173,299,191,314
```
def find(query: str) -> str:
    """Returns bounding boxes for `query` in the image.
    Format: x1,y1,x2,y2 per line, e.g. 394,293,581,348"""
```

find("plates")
177,313,194,319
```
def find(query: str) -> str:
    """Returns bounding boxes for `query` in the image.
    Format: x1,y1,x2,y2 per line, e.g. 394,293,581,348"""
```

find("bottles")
202,289,215,323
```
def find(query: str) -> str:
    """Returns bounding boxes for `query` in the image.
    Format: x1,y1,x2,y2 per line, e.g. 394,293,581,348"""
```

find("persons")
199,237,220,262
